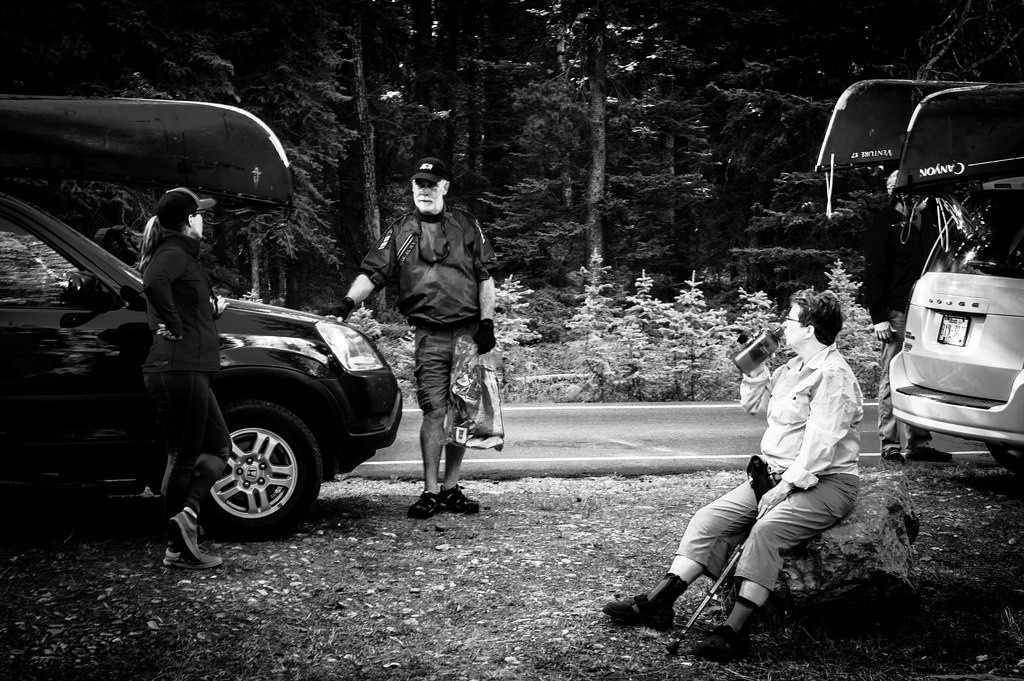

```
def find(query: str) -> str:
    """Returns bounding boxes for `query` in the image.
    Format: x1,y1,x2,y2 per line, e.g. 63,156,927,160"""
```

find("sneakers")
163,548,221,568
170,512,204,565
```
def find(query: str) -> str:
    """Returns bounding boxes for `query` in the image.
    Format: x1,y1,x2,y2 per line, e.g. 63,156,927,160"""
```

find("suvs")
1,191,404,542
886,176,1024,472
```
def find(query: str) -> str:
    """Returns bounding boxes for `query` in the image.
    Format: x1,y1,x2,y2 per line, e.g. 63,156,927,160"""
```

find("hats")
409,157,446,184
156,188,217,221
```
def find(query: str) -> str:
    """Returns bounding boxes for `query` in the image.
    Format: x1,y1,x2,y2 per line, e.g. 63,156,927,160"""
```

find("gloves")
472,319,496,355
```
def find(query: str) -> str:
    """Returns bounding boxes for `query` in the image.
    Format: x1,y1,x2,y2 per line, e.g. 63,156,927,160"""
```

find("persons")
317,156,498,519
144,187,230,569
864,169,952,464
602,288,863,656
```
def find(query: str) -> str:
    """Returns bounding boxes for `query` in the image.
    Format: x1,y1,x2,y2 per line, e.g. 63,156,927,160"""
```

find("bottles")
733,326,785,374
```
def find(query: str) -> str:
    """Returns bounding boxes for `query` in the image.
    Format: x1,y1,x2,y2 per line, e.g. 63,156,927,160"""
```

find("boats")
0,93,293,222
812,79,1005,174
894,85,1023,191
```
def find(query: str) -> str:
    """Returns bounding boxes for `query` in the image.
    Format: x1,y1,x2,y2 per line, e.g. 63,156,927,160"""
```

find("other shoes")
695,625,752,661
906,446,952,462
881,448,904,463
603,594,674,628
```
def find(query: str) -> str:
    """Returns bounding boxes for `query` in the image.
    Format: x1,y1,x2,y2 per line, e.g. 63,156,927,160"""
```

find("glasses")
186,209,208,223
780,314,807,326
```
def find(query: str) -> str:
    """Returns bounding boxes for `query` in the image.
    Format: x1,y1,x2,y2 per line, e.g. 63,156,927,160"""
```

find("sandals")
408,490,440,519
437,485,478,512
